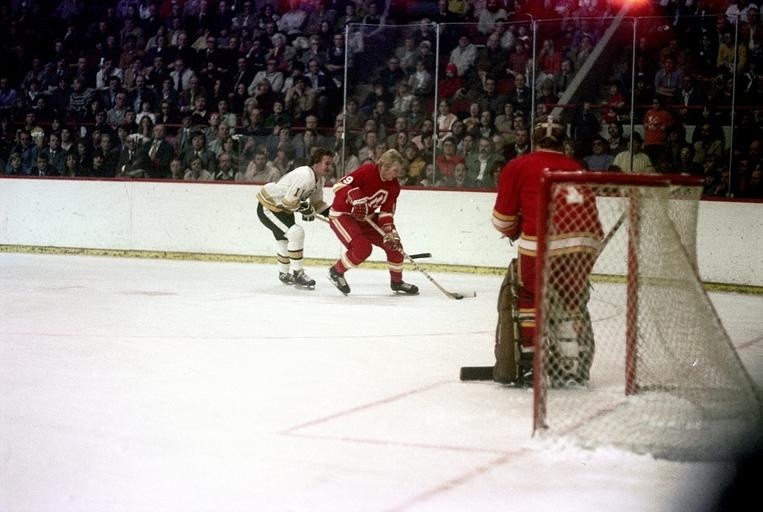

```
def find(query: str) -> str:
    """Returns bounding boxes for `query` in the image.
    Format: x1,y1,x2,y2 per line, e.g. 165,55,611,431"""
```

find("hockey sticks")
312,211,430,259
459,210,628,379
366,219,478,299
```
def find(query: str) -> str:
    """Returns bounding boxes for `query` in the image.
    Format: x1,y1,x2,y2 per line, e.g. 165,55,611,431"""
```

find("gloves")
298,202,316,222
381,223,401,251
347,187,373,220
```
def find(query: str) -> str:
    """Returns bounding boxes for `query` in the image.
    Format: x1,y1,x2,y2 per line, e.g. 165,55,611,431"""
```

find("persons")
328,148,420,297
2,1,624,189
562,0,762,202
489,117,602,387
255,150,335,289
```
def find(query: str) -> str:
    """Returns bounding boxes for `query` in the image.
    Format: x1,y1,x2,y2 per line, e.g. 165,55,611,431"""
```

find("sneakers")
279,271,294,283
292,269,316,286
328,265,351,294
389,280,419,294
